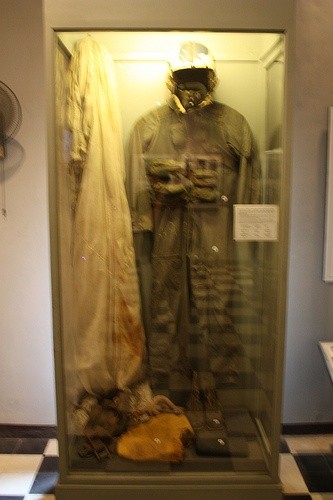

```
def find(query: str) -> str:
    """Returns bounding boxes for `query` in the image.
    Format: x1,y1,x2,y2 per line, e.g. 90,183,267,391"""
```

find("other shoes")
186,388,206,430
204,390,225,430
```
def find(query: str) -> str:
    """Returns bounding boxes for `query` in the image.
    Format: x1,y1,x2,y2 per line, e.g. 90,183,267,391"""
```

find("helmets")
169,42,216,87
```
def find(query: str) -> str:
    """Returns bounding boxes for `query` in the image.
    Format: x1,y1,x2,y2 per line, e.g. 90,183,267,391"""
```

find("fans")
0,79,23,161
